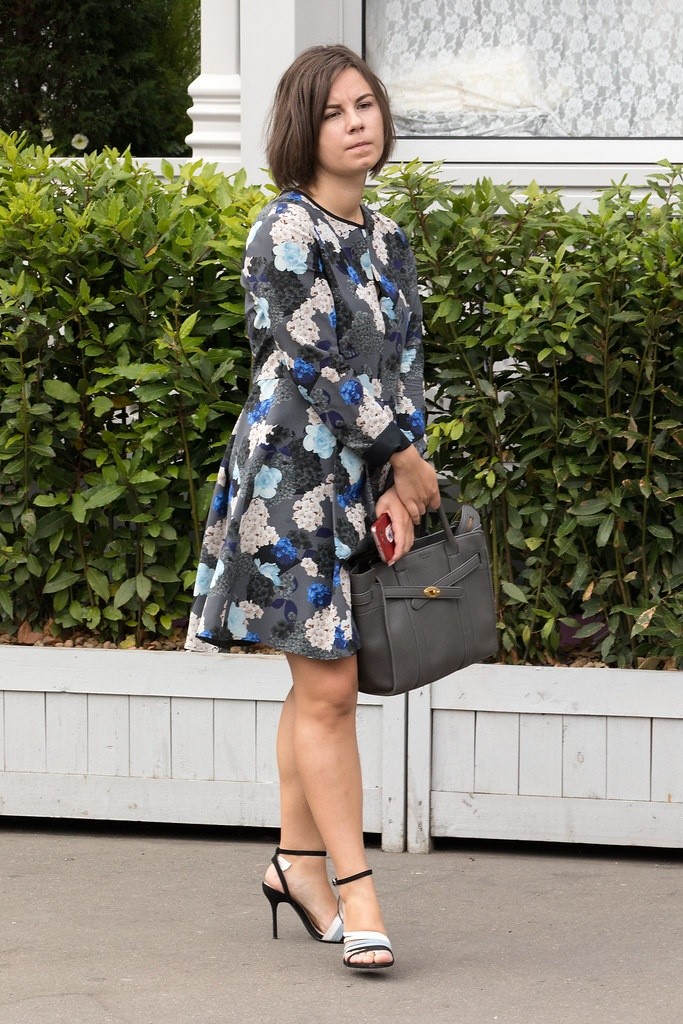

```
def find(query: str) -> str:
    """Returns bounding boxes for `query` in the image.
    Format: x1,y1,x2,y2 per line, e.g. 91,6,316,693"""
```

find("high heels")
262,848,345,945
332,869,395,971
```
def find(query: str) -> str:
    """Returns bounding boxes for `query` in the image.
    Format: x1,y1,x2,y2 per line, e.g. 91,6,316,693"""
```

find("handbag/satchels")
350,499,500,696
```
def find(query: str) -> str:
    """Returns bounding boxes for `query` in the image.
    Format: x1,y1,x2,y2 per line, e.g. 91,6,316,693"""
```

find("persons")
183,45,441,975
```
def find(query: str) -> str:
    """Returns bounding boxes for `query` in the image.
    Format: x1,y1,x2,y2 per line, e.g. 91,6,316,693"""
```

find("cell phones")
371,512,396,562
